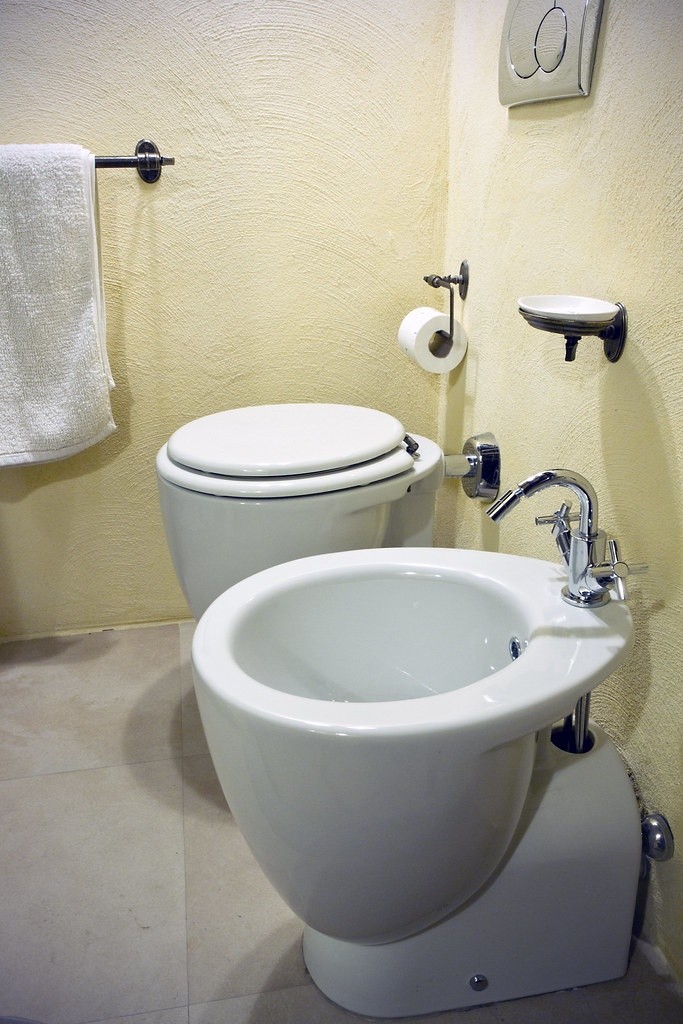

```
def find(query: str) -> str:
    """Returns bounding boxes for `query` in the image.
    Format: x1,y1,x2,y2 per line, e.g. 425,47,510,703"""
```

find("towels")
3,140,129,469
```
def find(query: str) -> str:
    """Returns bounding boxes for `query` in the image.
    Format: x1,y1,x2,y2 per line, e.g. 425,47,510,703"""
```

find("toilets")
156,404,444,625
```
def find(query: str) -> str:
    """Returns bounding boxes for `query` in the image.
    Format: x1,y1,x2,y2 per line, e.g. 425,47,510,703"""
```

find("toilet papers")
396,306,465,375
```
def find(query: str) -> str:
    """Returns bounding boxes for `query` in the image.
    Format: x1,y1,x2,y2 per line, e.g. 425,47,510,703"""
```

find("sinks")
189,543,635,948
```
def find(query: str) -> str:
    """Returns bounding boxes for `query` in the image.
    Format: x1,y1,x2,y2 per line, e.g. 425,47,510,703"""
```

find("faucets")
486,468,651,611
